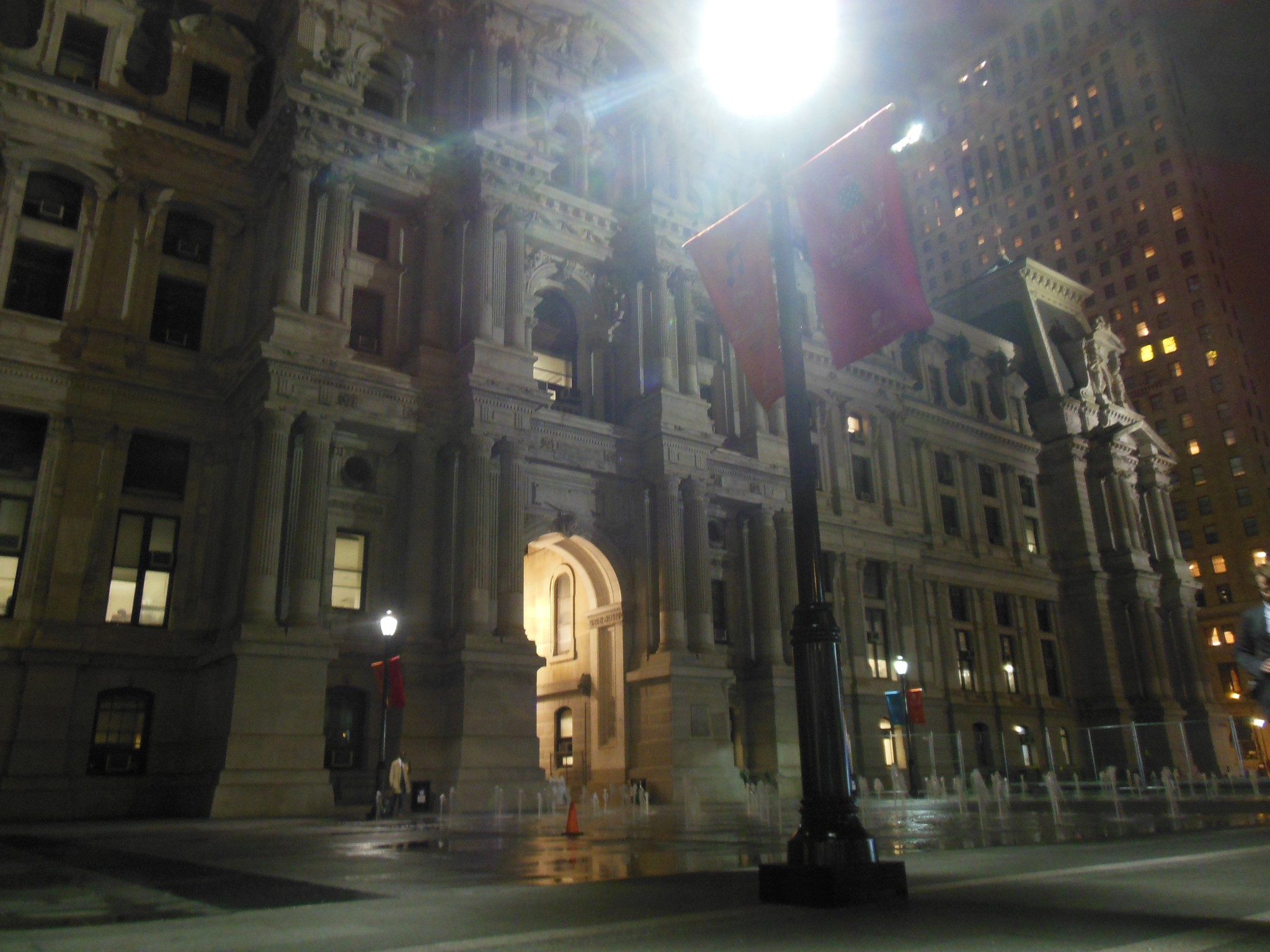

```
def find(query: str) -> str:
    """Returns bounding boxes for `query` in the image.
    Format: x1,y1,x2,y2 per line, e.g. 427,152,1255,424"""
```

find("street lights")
699,1,910,909
893,655,925,800
366,609,398,822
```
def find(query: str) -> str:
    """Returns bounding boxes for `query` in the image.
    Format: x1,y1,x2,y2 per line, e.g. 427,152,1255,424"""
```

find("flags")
907,691,926,724
689,199,786,409
372,659,406,708
887,693,905,725
791,118,932,370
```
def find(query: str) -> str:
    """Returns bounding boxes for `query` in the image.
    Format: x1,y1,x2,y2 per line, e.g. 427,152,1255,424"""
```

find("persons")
384,753,412,818
1111,349,1125,407
1089,357,1107,394
549,13,573,54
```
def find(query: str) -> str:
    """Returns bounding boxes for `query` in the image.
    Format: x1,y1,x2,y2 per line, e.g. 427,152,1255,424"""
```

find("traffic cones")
562,797,584,836
